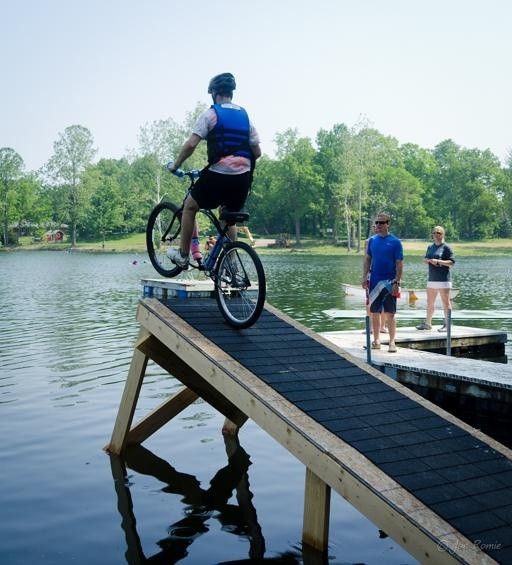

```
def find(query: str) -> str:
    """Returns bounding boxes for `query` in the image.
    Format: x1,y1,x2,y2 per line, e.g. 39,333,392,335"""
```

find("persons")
362,223,389,334
205,241,213,250
416,226,455,332
209,236,217,244
169,71,263,275
363,214,404,352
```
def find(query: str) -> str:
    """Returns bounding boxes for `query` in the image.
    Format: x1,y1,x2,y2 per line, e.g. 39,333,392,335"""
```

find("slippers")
363,342,380,349
388,344,397,352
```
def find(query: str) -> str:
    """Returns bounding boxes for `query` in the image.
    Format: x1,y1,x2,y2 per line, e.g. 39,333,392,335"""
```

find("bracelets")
436,259,440,266
393,281,401,285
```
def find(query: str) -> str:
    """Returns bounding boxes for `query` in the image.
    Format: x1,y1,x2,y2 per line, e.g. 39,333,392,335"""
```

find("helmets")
208,73,236,93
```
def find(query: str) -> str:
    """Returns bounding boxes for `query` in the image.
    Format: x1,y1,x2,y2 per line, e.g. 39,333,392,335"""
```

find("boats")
341,282,460,308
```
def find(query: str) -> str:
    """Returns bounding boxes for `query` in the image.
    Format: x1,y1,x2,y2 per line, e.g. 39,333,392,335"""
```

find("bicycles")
146,164,266,331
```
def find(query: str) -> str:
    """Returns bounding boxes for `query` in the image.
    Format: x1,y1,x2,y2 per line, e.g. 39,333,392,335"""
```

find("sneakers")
416,323,432,330
166,247,190,269
438,325,447,332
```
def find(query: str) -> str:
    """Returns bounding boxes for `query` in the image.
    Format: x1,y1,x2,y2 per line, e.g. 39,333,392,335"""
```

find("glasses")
433,232,440,234
375,220,389,225
